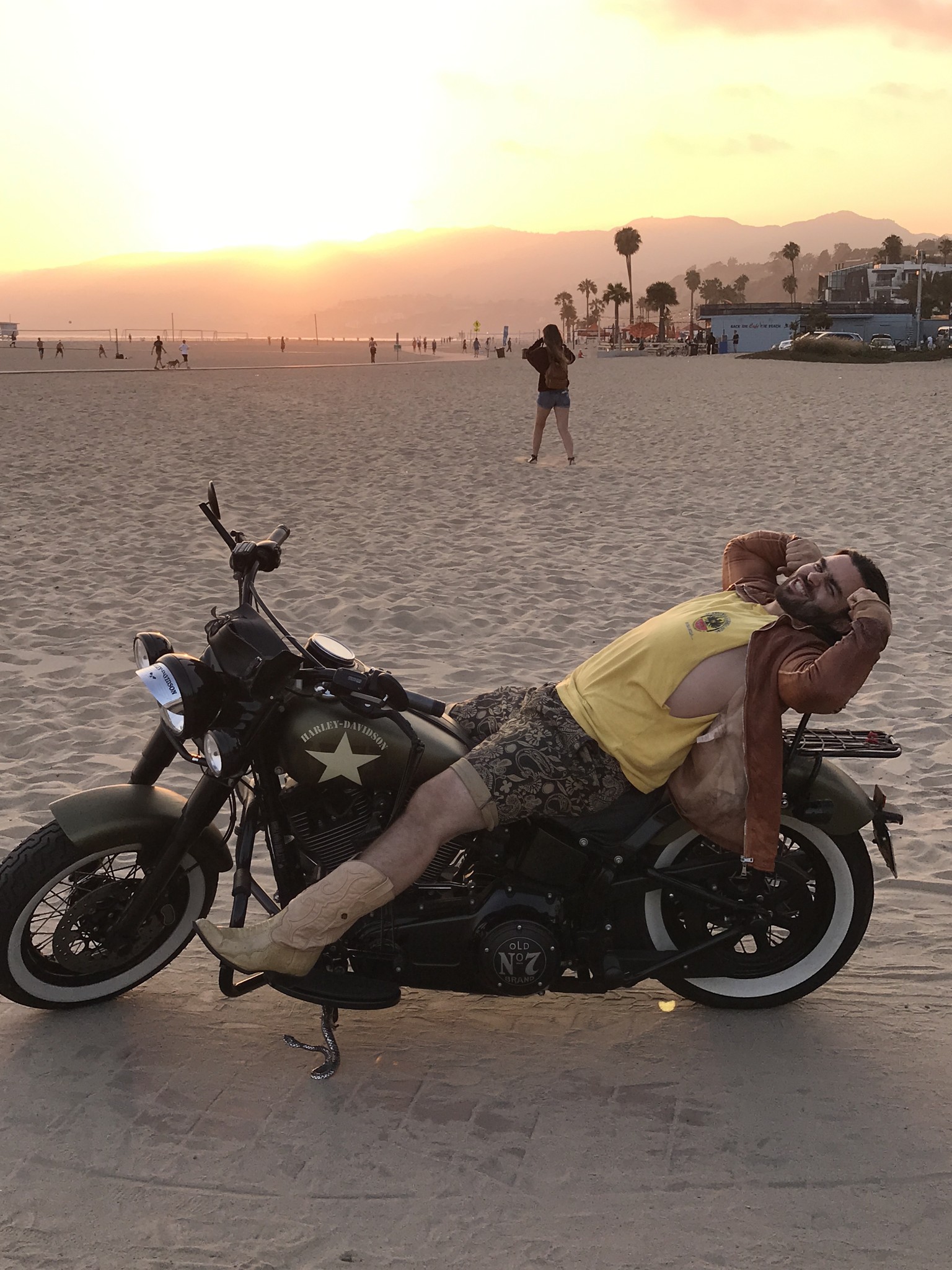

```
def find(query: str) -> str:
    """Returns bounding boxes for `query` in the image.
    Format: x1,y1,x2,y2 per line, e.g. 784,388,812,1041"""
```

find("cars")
869,333,910,351
783,332,864,350
937,326,952,340
779,331,825,350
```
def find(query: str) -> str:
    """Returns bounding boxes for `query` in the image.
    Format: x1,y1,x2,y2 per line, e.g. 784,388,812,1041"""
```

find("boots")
191,850,400,983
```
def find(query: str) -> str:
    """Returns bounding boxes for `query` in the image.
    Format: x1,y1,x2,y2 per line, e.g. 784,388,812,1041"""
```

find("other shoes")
153,366,159,370
526,454,538,464
187,366,191,369
162,365,165,369
178,363,180,368
371,360,375,363
567,456,576,465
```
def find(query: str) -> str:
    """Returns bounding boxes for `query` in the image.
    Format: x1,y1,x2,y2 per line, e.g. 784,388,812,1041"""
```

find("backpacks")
544,344,569,391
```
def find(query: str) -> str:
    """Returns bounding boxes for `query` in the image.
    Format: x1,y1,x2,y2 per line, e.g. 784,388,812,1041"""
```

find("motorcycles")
1,480,905,1083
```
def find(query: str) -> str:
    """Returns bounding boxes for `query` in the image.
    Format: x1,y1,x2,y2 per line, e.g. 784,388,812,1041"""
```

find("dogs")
166,359,180,369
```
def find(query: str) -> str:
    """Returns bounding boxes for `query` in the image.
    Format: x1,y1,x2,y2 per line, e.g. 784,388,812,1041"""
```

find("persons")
525,324,577,466
728,330,739,353
281,336,285,352
128,333,132,343
432,339,437,355
368,337,377,363
177,340,191,368
462,336,518,358
191,531,894,991
267,336,273,346
577,348,587,358
923,334,942,352
98,344,108,358
441,336,452,344
609,329,716,356
9,331,17,348
37,337,45,360
151,335,167,370
55,340,66,358
412,335,427,354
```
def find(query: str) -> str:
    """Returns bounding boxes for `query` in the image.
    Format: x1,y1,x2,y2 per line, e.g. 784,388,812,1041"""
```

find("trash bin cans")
496,347,505,358
522,348,528,359
689,344,699,356
